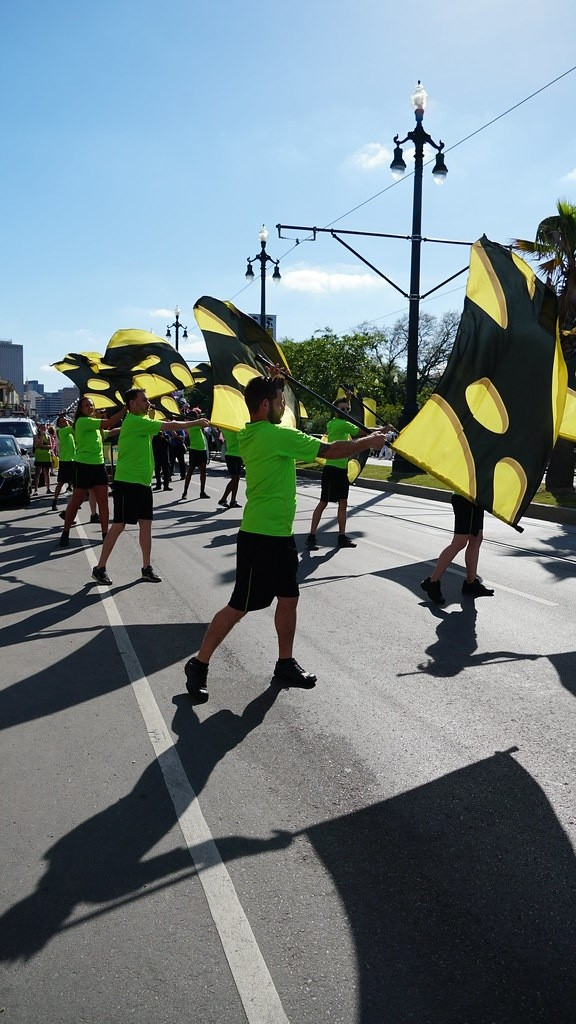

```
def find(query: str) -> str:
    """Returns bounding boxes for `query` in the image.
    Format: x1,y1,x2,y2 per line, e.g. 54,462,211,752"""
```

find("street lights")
167,305,189,352
245,224,283,334
389,78,450,474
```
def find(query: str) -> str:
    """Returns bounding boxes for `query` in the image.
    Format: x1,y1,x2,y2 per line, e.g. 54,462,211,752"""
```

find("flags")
192,295,305,434
144,395,181,420
393,232,570,527
100,328,195,399
49,352,124,408
190,363,212,383
558,390,576,441
314,386,377,484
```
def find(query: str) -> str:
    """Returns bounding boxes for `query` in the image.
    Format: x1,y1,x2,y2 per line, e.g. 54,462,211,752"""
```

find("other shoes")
46,489,53,494
164,486,173,491
230,501,242,508
200,492,210,498
153,484,161,490
59,510,65,519
52,501,58,511
218,499,230,508
182,492,187,499
90,513,101,522
60,530,69,547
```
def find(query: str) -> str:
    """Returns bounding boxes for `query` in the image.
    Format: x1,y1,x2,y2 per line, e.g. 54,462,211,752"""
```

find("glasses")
341,407,350,411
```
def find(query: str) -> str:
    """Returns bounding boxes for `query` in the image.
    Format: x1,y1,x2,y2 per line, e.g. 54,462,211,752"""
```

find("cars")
0,435,33,504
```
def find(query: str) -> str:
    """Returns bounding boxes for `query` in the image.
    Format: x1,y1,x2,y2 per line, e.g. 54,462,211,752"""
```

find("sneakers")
92,566,113,584
142,566,161,582
185,656,209,701
274,659,317,684
338,536,357,547
421,576,445,604
462,575,494,594
306,536,319,550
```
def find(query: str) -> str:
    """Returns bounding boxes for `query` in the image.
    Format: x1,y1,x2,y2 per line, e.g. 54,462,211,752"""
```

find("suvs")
0,417,43,485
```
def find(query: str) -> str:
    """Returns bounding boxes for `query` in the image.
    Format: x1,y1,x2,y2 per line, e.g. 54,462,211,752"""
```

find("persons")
92,387,210,585
33,397,244,546
185,365,387,701
304,389,394,551
420,491,494,605
